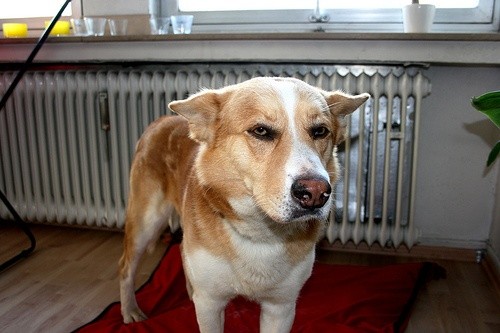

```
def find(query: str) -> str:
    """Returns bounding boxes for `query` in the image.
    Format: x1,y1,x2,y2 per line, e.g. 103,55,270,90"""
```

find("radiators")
0,69,431,251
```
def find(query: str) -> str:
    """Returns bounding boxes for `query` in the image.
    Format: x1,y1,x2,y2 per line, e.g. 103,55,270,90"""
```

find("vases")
402,4,435,34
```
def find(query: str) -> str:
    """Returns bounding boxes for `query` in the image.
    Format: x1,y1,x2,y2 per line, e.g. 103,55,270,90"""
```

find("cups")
403,4,435,32
46,21,68,36
171,15,193,34
83,17,106,36
70,19,83,36
3,24,28,38
149,17,170,34
108,19,128,35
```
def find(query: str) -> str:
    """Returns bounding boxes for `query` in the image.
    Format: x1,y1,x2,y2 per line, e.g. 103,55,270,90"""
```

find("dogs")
117,76,371,333
